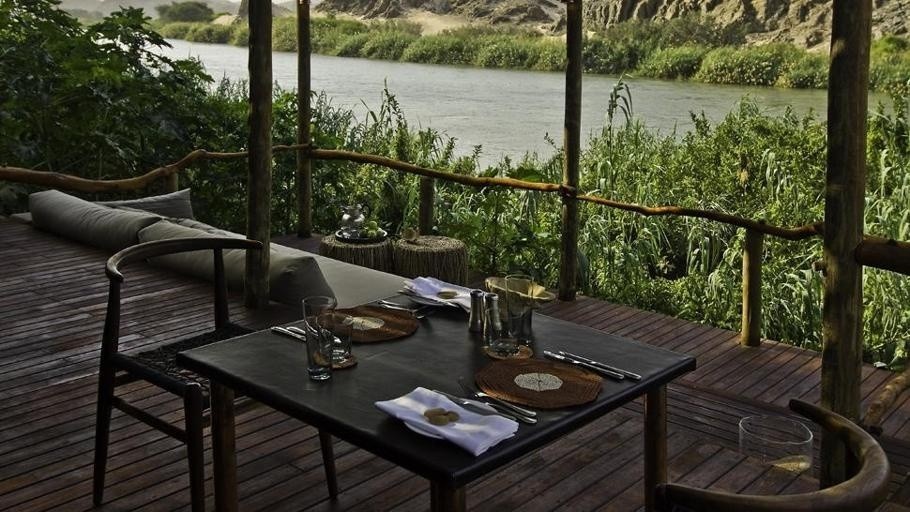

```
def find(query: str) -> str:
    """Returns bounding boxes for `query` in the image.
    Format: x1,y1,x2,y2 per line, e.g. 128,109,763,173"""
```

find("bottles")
484,293,502,335
469,290,483,332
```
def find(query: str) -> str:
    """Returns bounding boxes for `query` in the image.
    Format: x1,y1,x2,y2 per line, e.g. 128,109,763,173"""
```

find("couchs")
29,188,415,322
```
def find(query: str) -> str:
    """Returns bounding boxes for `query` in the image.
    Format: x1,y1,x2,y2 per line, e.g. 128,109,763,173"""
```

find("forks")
456,373,539,418
378,298,419,308
465,383,539,425
379,302,426,319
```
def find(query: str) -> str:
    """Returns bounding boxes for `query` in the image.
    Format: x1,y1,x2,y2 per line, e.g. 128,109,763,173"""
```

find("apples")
367,230,377,238
376,230,385,237
369,221,377,230
359,230,367,237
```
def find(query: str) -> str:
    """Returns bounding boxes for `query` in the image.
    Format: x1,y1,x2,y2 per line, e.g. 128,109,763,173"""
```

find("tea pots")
341,203,371,232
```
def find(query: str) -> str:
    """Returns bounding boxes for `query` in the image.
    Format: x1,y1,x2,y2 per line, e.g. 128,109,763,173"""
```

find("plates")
401,397,500,441
335,227,388,243
407,294,446,307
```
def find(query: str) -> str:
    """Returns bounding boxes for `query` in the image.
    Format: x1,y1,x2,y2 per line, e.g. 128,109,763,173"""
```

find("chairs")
654,398,893,512
87,235,346,512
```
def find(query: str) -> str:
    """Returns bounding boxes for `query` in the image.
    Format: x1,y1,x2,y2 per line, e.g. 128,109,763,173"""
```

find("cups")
481,305,510,357
504,275,534,344
302,295,338,382
317,314,354,363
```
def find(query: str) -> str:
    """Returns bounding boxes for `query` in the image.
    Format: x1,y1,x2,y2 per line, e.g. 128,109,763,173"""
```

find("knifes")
559,349,642,383
431,386,517,423
271,325,306,342
543,349,624,382
